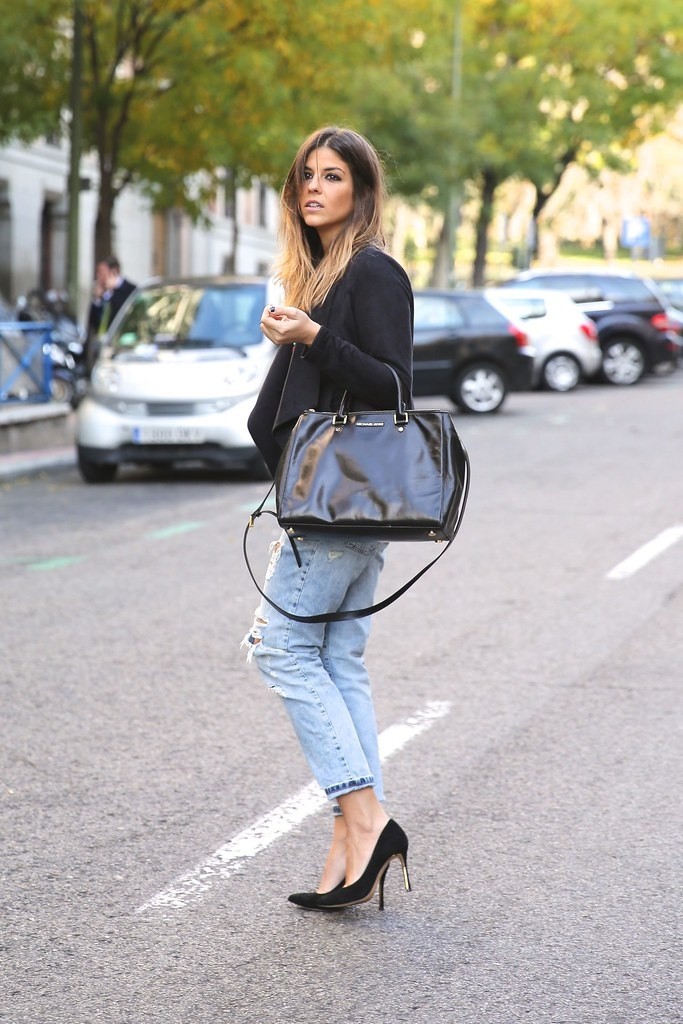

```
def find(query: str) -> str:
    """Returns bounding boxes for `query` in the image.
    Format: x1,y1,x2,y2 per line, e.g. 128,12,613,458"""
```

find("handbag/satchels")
242,362,471,623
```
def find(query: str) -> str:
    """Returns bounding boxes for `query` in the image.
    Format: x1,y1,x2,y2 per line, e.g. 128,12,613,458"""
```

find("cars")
477,290,603,393
406,289,533,415
491,272,683,386
73,273,290,484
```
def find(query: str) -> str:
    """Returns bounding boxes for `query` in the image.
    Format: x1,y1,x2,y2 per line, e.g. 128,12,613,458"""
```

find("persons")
244,128,413,909
19,290,78,374
71,255,145,410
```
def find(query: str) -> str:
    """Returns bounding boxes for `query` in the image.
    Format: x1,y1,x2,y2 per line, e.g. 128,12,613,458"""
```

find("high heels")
317,818,412,907
288,858,389,912
